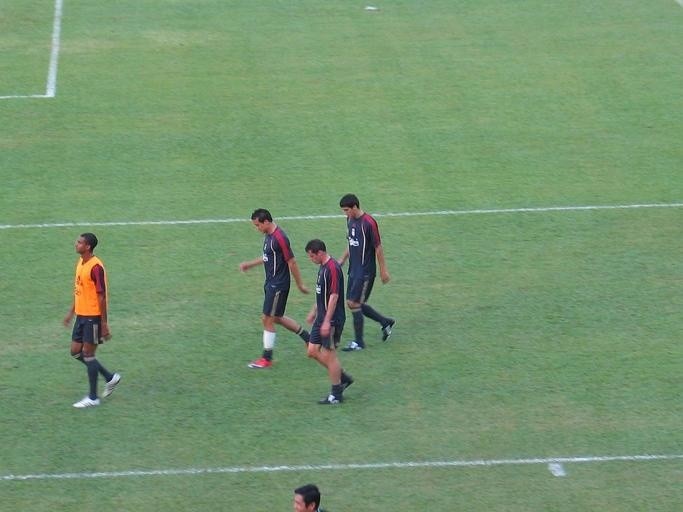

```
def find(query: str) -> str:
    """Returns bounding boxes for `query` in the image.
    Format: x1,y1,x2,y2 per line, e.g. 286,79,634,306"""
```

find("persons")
64,233,122,408
335,195,394,351
292,484,327,512
237,209,311,369
302,240,355,404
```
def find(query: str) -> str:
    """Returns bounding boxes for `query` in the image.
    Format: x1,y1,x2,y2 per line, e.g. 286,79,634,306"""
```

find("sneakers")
102,372,121,398
73,396,100,408
341,375,353,390
318,393,343,404
343,341,364,351
247,358,273,370
383,317,395,342
